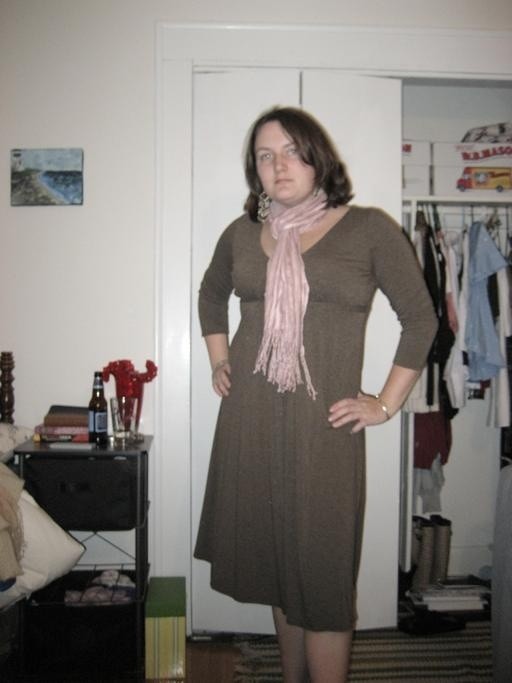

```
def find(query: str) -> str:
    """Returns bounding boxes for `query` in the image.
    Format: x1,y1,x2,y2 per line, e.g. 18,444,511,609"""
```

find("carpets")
234,618,494,681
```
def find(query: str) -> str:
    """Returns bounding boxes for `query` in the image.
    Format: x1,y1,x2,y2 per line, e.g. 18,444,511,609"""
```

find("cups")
111,396,138,451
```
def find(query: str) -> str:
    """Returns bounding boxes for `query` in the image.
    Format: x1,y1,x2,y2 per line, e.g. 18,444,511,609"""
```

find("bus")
456,166,512,192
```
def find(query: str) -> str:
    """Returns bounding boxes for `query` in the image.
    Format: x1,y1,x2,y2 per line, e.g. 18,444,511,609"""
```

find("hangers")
407,204,510,247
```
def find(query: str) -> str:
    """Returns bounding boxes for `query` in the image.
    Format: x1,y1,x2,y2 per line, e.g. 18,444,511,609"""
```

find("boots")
412,514,451,590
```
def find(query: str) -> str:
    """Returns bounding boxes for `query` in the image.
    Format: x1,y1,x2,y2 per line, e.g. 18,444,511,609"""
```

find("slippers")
399,613,465,635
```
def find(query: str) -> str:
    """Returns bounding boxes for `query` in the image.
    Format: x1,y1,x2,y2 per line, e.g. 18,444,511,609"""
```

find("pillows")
0,422,36,465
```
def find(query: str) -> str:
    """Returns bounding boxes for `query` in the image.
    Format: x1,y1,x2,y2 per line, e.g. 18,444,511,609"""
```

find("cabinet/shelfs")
192,71,508,633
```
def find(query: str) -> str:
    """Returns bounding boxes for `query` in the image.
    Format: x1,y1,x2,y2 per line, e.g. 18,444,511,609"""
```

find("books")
32,404,90,443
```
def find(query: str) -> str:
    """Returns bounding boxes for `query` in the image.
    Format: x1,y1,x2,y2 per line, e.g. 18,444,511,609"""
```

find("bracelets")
375,394,391,420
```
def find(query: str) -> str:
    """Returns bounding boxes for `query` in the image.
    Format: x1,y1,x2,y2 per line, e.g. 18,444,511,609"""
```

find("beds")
1,353,86,613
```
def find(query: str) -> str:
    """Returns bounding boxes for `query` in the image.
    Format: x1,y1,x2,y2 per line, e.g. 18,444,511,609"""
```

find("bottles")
87,371,108,450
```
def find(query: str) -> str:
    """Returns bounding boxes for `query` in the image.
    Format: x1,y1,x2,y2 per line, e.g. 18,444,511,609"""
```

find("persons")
194,106,441,683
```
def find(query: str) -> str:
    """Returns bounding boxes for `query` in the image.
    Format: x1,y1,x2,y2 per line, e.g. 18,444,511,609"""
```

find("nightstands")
17,441,148,680
